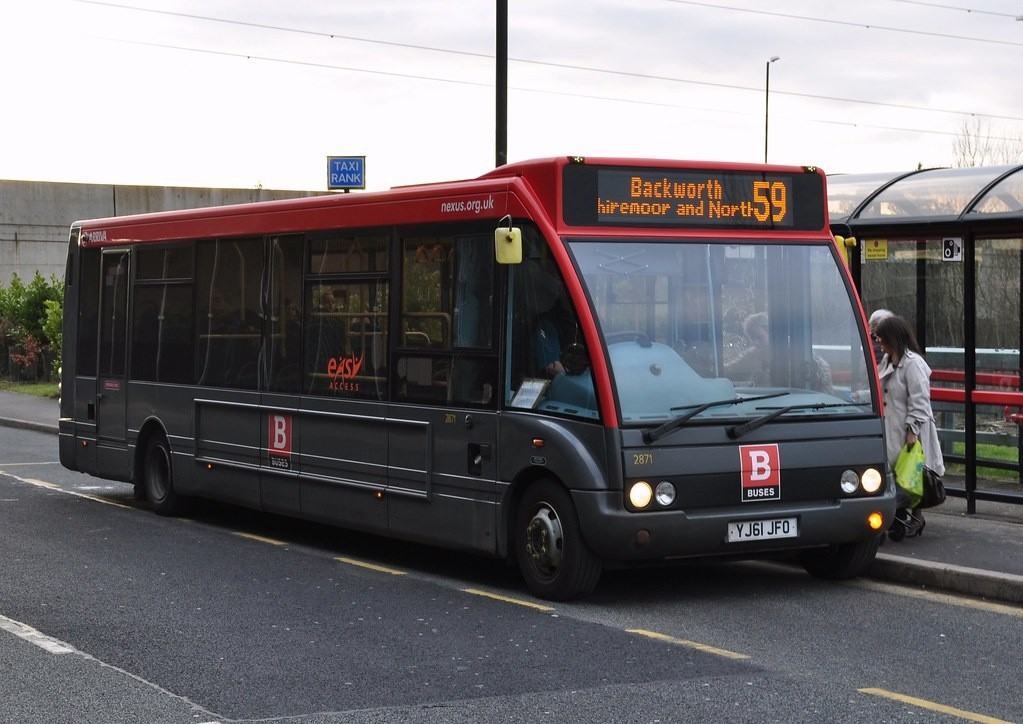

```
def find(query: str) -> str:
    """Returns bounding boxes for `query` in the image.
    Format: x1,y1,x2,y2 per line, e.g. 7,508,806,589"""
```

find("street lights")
761,53,785,163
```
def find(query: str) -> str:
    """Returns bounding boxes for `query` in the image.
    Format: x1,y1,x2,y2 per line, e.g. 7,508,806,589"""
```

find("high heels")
906,519,926,537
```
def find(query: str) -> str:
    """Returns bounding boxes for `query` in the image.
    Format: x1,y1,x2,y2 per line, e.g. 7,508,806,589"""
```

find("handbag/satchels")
912,464,946,511
895,440,924,507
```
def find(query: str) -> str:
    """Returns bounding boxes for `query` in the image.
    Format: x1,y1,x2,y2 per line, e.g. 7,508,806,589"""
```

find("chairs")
316,320,391,387
479,269,492,347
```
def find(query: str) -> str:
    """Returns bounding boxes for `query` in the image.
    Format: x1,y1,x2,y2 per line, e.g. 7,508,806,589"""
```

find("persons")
309,293,342,345
715,313,819,396
284,301,302,329
868,309,900,525
203,288,235,331
520,269,607,383
873,317,944,543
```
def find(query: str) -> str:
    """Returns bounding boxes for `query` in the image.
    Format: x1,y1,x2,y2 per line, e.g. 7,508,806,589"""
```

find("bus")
54,152,901,606
